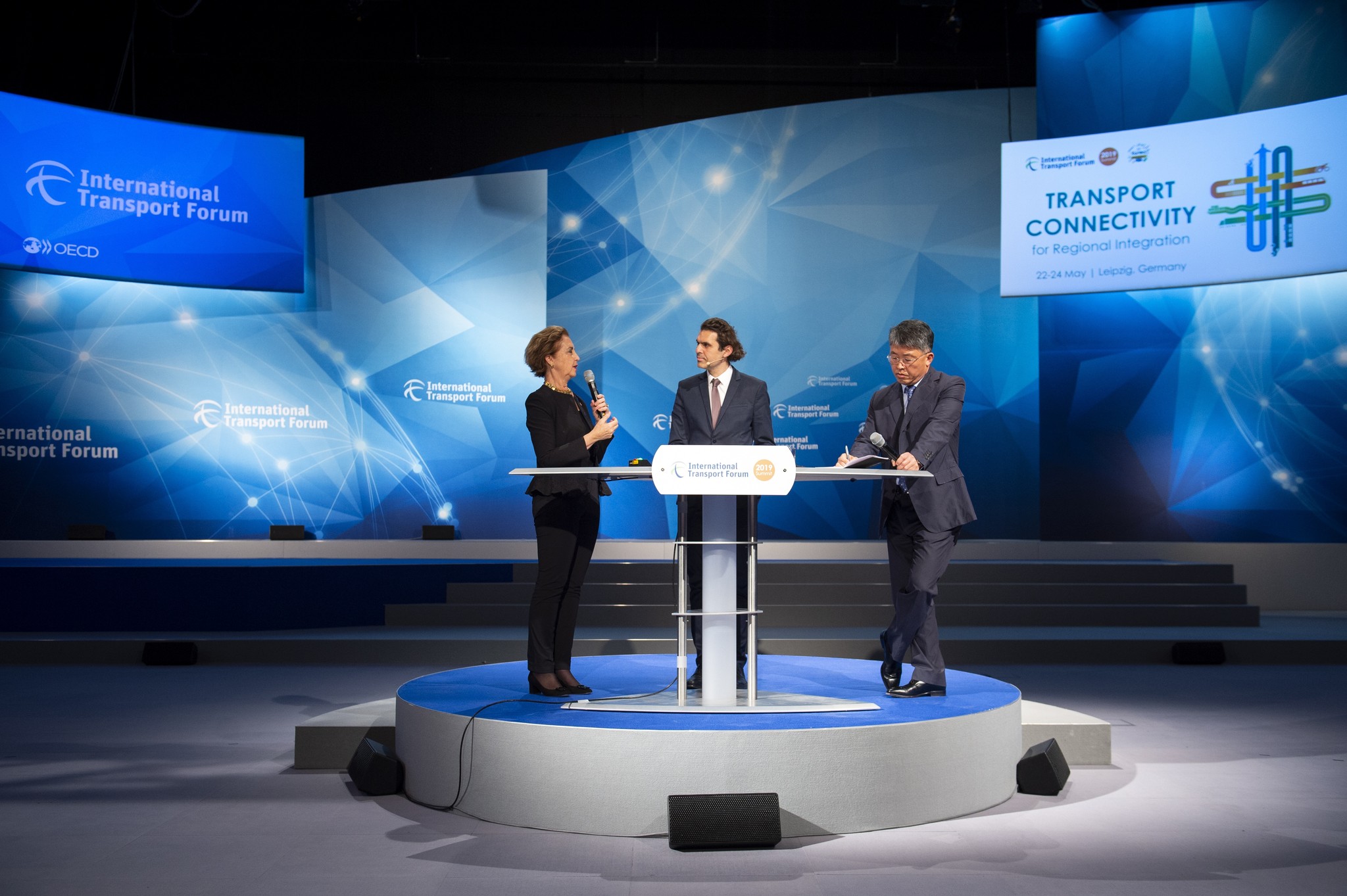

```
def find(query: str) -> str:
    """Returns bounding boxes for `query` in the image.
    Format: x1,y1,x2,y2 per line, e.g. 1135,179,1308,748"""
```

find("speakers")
667,792,781,849
422,525,455,540
269,525,304,540
348,738,406,796
67,525,106,540
1016,737,1070,795
141,640,197,666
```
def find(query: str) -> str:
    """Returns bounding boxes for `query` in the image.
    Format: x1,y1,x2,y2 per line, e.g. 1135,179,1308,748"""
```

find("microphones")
869,432,900,462
706,357,725,365
584,370,605,419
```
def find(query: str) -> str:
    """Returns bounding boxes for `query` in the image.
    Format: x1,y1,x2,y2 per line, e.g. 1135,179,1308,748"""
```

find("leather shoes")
687,664,702,689
885,679,946,698
736,667,748,689
879,629,902,690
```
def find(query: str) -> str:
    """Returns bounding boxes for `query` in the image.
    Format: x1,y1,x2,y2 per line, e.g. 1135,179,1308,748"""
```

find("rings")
606,404,609,407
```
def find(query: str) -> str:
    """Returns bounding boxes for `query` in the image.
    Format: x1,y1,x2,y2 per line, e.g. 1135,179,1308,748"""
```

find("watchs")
915,458,923,468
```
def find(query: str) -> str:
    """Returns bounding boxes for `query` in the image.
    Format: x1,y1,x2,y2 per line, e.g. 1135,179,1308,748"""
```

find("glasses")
886,352,931,366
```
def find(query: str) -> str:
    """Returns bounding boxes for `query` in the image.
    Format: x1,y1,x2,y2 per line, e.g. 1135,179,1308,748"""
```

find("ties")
898,385,916,493
711,379,722,431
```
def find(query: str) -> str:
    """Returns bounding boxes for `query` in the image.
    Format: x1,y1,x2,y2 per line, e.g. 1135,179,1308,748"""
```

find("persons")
666,317,776,691
524,325,620,697
834,319,976,701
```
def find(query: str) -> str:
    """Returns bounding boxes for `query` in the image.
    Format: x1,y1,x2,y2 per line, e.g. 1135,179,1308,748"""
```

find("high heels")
554,673,592,695
528,671,572,697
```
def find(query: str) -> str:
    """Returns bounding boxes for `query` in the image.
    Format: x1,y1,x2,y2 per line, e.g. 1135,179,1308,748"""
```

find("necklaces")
544,381,572,393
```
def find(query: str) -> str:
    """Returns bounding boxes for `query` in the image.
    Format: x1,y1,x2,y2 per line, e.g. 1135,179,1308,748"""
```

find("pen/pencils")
845,445,850,462
